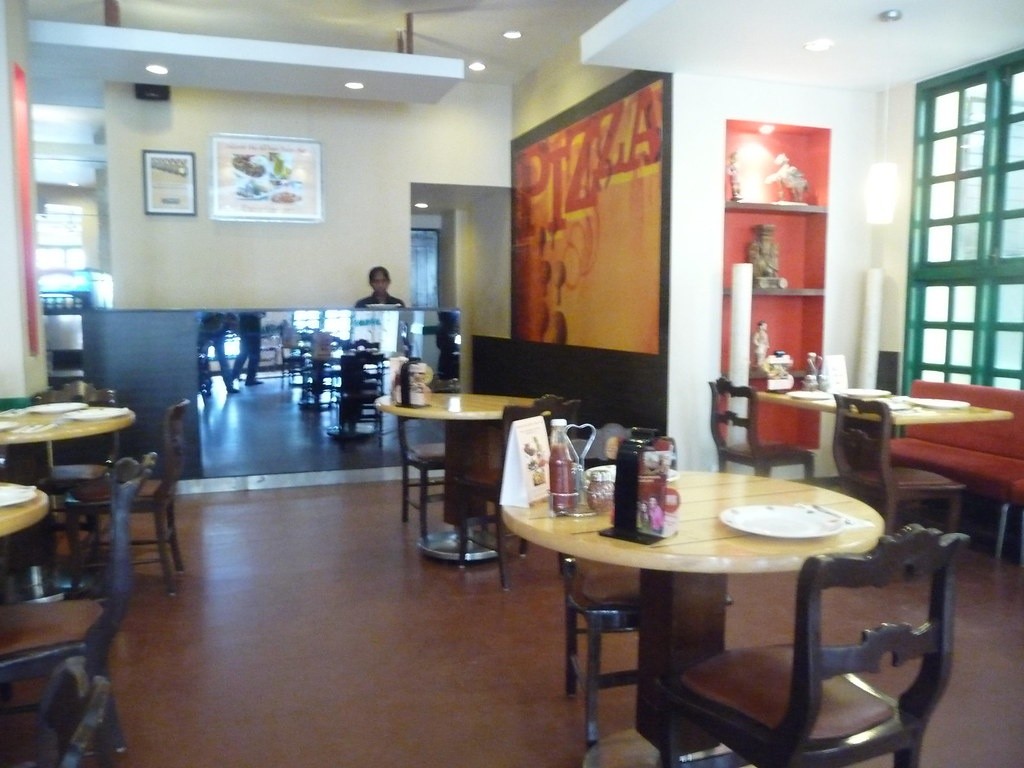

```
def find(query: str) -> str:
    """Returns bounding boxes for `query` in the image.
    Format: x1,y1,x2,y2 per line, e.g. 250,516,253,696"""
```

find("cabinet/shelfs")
719,119,831,454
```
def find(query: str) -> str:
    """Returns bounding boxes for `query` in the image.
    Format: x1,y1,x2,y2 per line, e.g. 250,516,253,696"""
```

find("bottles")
800,375,828,392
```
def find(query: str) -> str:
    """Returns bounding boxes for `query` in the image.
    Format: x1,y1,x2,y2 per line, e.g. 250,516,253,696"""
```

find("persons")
198,312,266,396
752,319,770,366
747,224,779,284
436,312,460,380
727,152,744,201
355,267,404,306
636,495,663,535
764,154,807,202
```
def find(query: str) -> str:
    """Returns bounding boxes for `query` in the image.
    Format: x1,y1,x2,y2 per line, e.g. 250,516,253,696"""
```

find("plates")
719,505,841,537
905,398,969,408
64,407,130,421
0,422,17,430
27,403,87,413
843,389,890,398
787,391,830,399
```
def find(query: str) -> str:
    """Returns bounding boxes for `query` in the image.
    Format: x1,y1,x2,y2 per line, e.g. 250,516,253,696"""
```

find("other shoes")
245,379,263,385
232,374,243,381
203,390,211,396
227,386,240,393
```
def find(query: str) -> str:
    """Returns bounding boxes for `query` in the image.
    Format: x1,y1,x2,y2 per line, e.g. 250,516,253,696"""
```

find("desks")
0,401,137,601
373,393,539,564
307,349,391,440
0,481,49,542
499,470,885,768
755,388,1015,428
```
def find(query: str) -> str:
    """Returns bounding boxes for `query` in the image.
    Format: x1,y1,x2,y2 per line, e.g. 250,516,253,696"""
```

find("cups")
550,419,575,514
448,398,460,412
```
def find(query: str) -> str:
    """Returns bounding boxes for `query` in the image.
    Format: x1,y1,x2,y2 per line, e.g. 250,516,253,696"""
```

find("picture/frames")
208,132,325,225
142,150,198,216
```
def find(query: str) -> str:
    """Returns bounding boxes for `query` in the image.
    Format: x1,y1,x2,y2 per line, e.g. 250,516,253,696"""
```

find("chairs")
834,392,969,576
280,327,389,449
559,421,736,747
708,377,815,485
453,392,583,590
394,368,461,532
0,380,198,768
651,524,971,768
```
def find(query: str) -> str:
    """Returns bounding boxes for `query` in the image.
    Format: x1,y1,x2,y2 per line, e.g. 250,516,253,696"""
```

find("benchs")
886,380,1024,558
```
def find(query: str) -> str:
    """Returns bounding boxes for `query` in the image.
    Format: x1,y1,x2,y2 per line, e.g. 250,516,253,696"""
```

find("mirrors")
198,308,463,479
